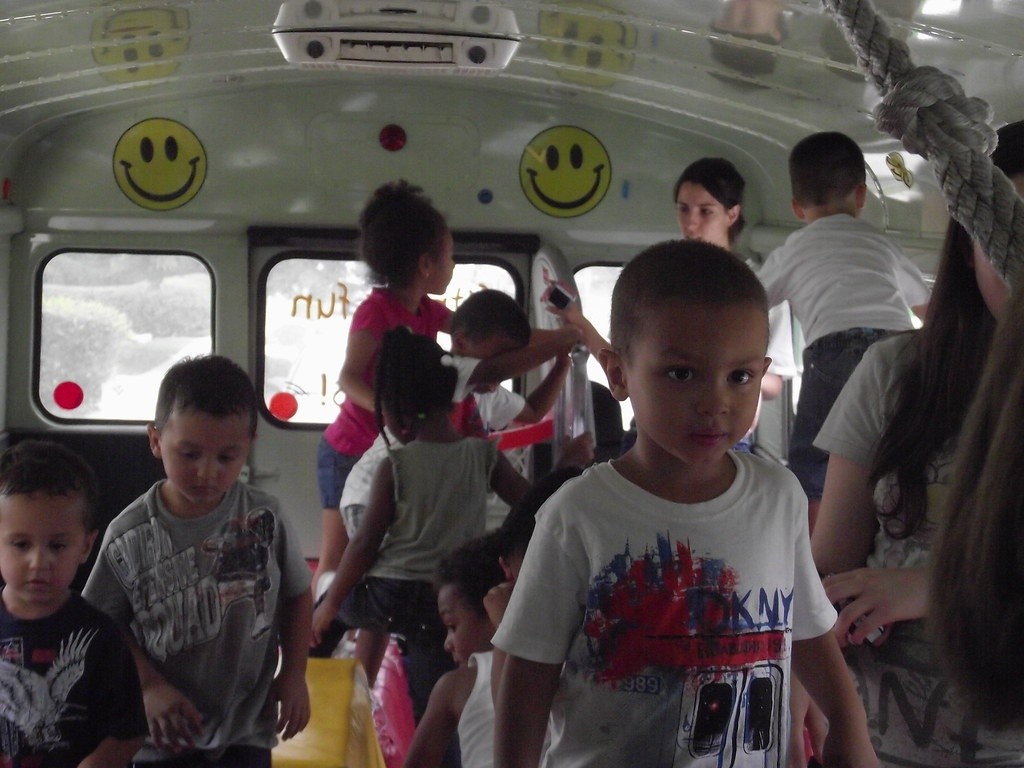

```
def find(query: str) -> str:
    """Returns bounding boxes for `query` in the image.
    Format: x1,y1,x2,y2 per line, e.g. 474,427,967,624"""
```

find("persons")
0,441,150,768
785,121,1023,768
758,130,932,536
541,157,793,453
311,180,582,687
80,355,312,768
491,240,881,767
400,495,554,768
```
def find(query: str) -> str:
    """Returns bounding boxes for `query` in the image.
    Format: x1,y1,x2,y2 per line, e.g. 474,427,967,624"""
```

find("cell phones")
547,284,577,311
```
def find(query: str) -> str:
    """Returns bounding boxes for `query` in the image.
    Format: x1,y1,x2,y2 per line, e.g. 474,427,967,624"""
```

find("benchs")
0,428,169,568
